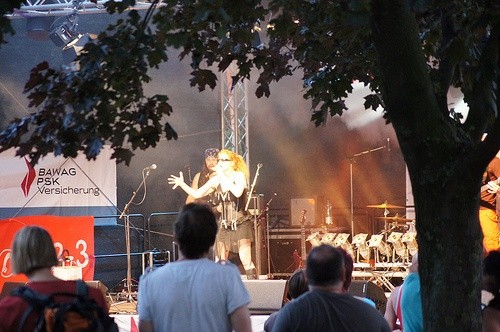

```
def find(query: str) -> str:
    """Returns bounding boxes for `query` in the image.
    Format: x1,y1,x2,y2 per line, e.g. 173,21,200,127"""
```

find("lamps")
305,228,417,267
50,3,86,47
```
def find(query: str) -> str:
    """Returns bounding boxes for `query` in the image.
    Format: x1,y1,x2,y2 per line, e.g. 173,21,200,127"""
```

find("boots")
246,269,258,280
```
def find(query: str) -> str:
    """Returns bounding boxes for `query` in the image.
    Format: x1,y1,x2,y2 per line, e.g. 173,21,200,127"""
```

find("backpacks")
10,279,116,332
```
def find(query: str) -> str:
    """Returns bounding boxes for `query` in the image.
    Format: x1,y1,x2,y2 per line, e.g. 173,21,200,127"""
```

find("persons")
264,244,393,332
138,201,253,332
479,167,500,252
384,248,500,332
168,148,258,280
0,227,120,332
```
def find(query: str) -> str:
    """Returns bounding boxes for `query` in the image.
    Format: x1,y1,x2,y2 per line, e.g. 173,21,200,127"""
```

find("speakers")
348,280,387,316
228,197,268,275
241,279,289,314
288,195,325,227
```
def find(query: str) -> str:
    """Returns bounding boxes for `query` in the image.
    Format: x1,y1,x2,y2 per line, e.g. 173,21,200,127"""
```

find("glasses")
217,158,232,162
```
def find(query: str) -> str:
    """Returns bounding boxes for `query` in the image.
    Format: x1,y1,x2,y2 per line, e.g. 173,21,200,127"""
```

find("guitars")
481,177,500,192
293,210,307,271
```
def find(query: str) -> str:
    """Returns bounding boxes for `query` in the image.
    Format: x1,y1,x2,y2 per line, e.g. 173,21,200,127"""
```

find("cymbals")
376,216,412,222
366,204,405,209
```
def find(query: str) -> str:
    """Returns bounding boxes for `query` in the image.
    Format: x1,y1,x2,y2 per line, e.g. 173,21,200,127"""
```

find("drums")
377,231,400,262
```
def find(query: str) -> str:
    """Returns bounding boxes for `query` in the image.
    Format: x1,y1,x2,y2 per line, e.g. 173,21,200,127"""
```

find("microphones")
144,164,157,170
387,137,391,151
